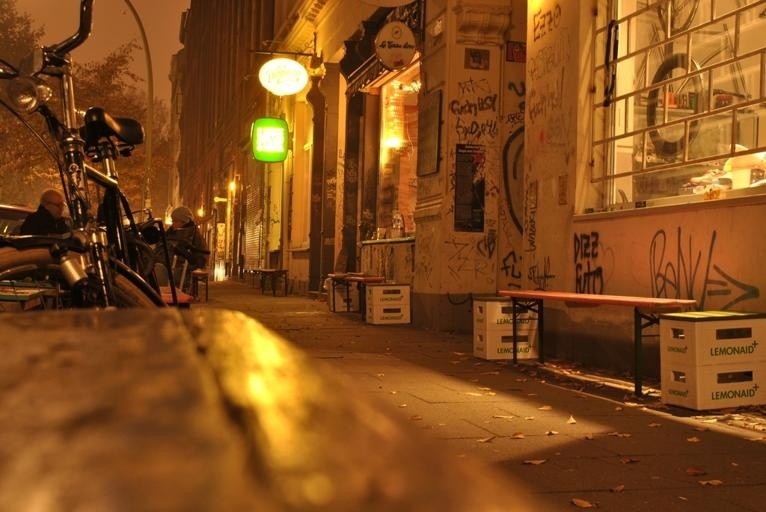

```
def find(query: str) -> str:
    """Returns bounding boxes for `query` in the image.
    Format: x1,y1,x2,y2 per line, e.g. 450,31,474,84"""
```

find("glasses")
44,201,66,210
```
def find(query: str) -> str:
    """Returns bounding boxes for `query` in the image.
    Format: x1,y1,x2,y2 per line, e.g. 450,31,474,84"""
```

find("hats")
171,206,193,224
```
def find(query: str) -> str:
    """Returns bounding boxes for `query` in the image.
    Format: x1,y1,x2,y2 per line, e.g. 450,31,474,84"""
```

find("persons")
151,204,209,287
15,187,82,287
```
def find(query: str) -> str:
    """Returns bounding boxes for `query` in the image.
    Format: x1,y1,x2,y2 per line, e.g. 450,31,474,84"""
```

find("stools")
192,270,209,304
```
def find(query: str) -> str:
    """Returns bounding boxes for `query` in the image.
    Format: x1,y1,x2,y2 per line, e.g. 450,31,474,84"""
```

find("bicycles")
0,0,177,313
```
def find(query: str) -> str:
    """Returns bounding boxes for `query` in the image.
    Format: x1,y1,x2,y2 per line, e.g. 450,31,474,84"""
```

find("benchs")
160,286,194,308
0,308,537,512
254,270,288,297
328,274,386,321
500,289,697,399
